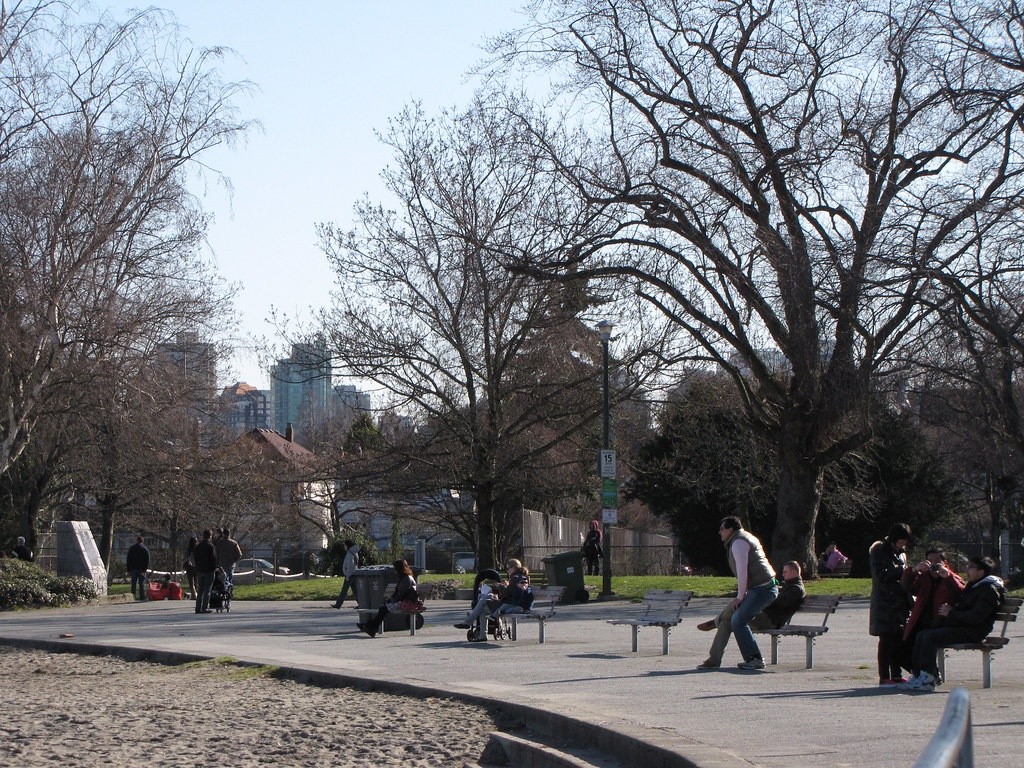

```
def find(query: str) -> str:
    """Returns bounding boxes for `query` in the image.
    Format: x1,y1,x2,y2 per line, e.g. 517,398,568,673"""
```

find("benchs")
745,594,843,669
609,589,696,655
802,558,853,578
899,597,1023,688
466,583,567,644
354,583,434,636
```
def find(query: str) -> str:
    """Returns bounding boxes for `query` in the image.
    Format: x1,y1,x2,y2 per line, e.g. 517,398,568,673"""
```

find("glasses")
506,567,511,571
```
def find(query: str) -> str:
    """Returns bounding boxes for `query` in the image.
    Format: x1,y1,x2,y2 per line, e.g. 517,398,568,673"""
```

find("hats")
518,576,528,583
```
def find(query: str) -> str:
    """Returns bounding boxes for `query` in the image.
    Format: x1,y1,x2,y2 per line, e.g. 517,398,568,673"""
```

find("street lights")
595,320,619,597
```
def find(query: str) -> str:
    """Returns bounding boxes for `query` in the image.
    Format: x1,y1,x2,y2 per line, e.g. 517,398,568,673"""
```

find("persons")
331,540,361,609
8,536,34,562
695,561,806,670
901,556,1007,692
583,520,602,576
718,516,778,670
818,543,849,574
185,527,242,614
127,536,150,600
356,559,420,637
161,573,171,590
868,523,913,687
900,548,965,685
454,559,534,642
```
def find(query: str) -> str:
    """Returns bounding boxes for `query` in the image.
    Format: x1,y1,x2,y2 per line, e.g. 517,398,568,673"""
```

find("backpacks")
349,550,366,567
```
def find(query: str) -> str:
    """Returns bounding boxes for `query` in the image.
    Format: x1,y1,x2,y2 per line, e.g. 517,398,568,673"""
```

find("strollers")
209,565,233,613
466,569,512,641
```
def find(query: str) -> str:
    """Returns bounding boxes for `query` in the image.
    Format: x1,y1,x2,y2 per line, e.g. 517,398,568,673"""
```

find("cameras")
929,562,941,570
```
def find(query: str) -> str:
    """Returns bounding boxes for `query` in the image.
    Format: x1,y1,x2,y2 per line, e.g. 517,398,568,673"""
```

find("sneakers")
897,669,935,691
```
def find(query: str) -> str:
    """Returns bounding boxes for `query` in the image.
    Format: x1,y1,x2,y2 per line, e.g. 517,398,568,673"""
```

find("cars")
454,552,475,572
235,559,292,575
943,552,972,572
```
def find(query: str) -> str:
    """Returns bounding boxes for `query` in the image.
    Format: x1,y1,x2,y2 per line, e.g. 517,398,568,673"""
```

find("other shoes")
473,637,487,642
879,677,909,688
202,610,212,613
499,609,506,614
454,623,471,629
355,622,375,638
697,619,716,631
486,615,496,622
330,604,340,609
737,656,766,670
697,658,721,669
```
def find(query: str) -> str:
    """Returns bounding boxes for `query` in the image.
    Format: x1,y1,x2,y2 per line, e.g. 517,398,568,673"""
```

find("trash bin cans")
352,564,424,631
542,550,590,604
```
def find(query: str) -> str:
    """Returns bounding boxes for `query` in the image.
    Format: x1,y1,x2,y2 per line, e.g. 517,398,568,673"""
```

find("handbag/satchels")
400,597,425,610
182,556,195,571
